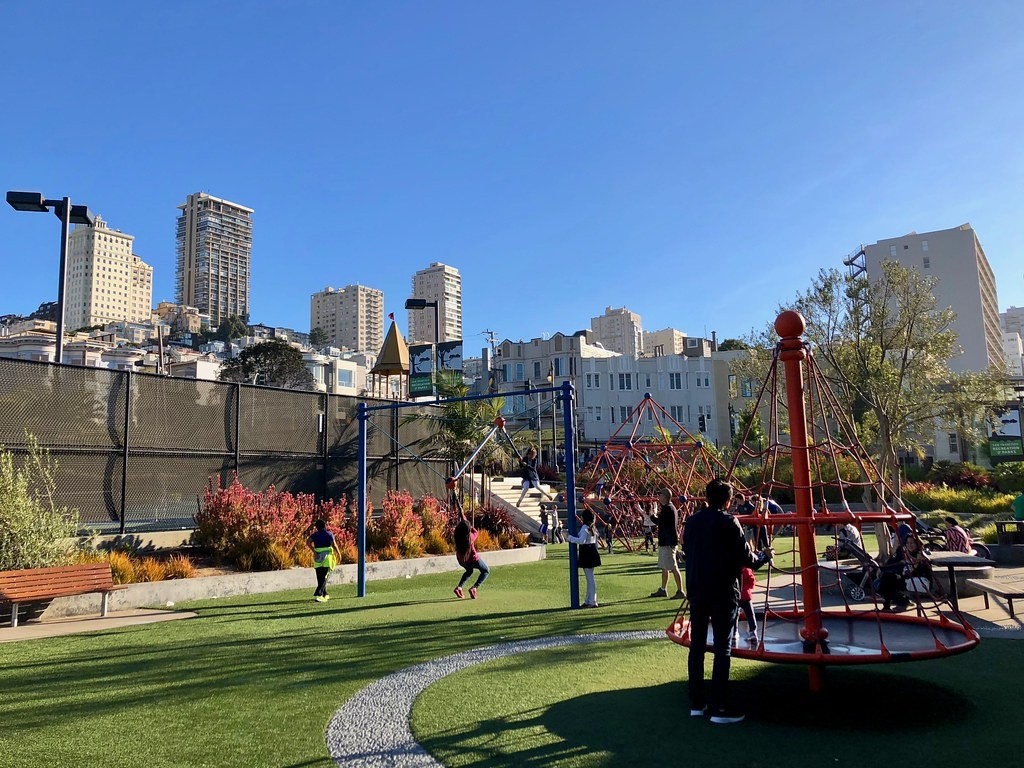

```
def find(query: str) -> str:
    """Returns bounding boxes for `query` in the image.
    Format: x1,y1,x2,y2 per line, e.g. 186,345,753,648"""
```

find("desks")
922,550,995,615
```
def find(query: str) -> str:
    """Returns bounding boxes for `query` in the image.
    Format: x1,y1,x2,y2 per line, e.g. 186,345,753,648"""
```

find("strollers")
832,528,879,602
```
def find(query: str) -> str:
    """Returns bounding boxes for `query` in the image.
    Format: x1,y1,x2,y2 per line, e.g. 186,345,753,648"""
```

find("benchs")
966,578,1024,619
0,561,128,628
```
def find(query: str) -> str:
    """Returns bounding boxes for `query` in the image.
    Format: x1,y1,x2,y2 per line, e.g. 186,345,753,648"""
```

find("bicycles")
922,532,991,561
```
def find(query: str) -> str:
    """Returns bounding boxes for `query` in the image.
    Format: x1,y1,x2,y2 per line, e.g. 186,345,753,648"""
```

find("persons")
873,523,932,613
563,509,602,608
306,519,341,602
647,488,687,600
733,492,786,551
826,523,863,560
539,503,565,544
943,517,975,556
605,523,613,554
733,565,759,641
515,447,553,507
682,479,774,724
454,509,490,599
636,502,658,551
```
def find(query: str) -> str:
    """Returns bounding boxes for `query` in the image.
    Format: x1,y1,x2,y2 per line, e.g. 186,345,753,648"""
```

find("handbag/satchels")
665,597,692,647
905,576,930,593
826,546,841,561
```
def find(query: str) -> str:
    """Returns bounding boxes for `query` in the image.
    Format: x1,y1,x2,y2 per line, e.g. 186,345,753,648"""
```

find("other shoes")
581,603,597,608
732,632,740,640
892,605,906,613
748,632,758,641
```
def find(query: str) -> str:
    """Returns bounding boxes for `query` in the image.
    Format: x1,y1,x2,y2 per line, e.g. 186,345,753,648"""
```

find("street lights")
405,299,440,402
548,368,559,471
5,191,95,508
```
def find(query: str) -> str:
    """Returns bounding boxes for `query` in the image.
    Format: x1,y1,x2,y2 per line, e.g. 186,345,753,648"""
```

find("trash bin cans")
996,521,1024,544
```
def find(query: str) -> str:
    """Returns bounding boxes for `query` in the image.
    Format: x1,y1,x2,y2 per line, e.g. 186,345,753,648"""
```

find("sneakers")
669,591,686,599
709,709,746,724
469,586,477,600
651,587,668,597
315,594,330,603
454,586,465,599
690,704,708,716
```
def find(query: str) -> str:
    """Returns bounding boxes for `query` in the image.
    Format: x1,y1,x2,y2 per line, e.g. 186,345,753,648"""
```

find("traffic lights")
698,414,707,432
524,379,532,399
